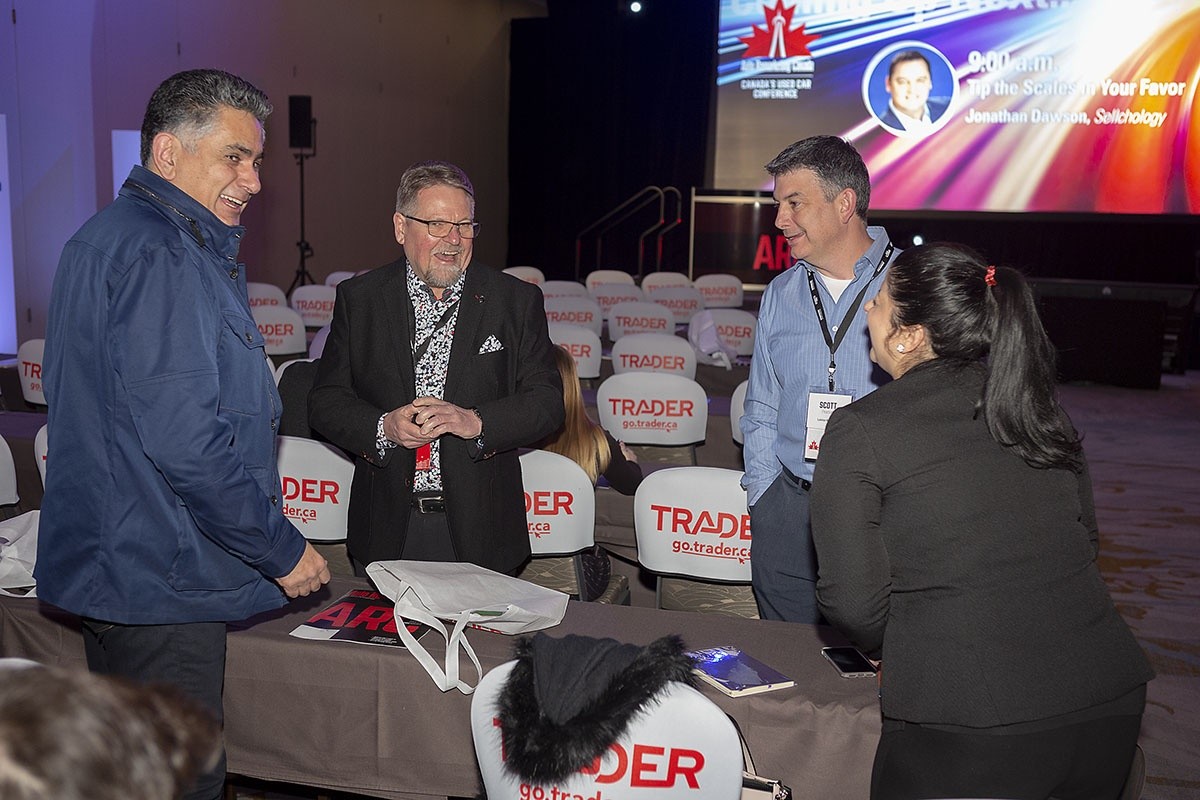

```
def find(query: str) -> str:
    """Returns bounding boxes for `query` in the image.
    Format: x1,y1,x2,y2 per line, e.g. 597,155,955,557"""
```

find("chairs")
0,264,761,619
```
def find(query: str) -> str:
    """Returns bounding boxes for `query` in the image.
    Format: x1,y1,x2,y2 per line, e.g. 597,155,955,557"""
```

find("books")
682,646,795,698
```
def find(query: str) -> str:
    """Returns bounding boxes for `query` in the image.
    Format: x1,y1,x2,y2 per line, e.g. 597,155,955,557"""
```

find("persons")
313,160,643,601
812,243,1154,800
881,52,946,132
741,135,907,624
0,69,330,800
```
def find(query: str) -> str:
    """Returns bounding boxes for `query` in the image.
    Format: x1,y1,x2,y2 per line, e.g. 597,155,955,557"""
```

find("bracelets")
470,408,485,440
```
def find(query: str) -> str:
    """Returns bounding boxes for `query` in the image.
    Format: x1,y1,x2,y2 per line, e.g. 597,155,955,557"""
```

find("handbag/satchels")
365,560,570,695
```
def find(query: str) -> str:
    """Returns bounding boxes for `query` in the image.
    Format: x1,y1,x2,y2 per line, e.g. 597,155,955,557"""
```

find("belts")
413,496,446,515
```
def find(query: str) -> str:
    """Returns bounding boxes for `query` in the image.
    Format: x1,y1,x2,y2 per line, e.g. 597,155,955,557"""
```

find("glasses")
400,213,482,239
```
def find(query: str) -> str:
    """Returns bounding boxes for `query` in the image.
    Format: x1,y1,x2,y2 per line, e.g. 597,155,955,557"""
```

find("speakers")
287,93,312,148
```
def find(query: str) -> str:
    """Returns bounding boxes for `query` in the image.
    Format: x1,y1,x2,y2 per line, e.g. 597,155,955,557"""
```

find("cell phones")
819,644,879,680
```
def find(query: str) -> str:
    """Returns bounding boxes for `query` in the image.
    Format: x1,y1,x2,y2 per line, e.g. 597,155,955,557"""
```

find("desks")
0,567,879,800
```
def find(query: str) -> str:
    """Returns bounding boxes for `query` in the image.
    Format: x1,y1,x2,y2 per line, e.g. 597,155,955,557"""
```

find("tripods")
284,154,317,299
